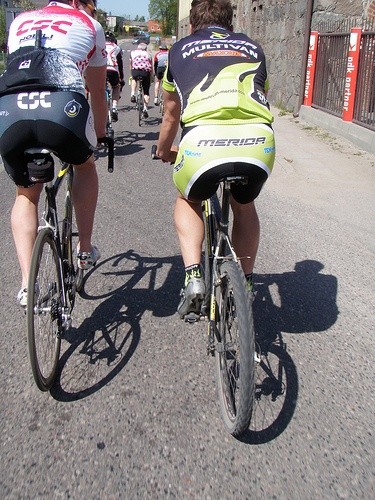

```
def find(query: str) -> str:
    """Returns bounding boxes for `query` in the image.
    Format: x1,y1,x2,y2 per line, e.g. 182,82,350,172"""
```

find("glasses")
80,0,98,17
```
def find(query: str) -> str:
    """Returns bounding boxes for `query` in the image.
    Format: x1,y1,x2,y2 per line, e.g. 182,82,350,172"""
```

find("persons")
130,42,153,118
104,30,125,123
155,0,274,320
154,43,171,106
1,0,108,308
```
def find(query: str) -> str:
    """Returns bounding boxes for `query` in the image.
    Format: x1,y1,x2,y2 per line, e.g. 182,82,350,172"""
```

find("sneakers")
16,282,40,308
77,245,101,271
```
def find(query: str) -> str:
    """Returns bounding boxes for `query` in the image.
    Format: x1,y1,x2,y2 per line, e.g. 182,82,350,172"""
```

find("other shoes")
130,95,136,103
143,109,149,119
176,278,205,319
154,99,158,106
112,108,119,120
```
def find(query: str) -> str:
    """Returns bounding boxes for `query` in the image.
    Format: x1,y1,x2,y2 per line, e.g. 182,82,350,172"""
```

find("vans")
131,35,150,45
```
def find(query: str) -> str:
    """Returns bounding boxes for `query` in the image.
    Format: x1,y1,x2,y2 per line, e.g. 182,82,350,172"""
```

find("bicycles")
128,75,151,126
150,144,256,439
105,76,125,122
154,73,165,116
25,127,116,393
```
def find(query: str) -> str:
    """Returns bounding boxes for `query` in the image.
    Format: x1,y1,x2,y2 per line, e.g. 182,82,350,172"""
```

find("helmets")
138,43,147,50
158,45,167,49
105,31,116,40
